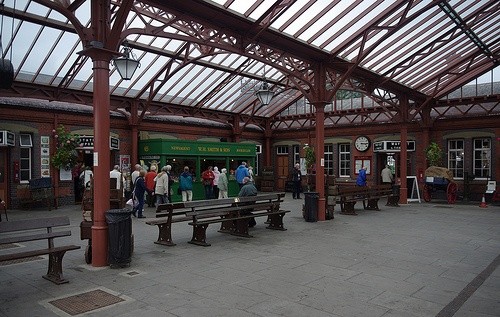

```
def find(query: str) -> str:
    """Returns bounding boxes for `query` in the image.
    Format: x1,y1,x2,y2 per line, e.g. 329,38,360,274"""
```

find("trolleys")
422,167,464,205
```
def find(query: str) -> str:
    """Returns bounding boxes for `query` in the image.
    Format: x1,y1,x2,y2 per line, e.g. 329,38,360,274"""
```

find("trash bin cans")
392,185,400,199
304,191,320,222
105,208,133,265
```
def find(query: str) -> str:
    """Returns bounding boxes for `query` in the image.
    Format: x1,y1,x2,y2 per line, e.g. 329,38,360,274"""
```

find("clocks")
353,135,371,153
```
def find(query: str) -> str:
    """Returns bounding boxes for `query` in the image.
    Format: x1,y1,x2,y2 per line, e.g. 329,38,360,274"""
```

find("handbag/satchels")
125,199,133,207
177,188,182,195
79,170,85,188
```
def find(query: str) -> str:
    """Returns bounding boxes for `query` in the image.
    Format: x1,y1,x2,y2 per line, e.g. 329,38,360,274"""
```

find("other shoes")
138,215,146,218
297,197,302,199
132,211,136,217
248,221,256,228
293,197,297,199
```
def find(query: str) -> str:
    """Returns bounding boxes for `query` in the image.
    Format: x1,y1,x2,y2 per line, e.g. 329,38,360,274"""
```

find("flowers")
51,126,81,170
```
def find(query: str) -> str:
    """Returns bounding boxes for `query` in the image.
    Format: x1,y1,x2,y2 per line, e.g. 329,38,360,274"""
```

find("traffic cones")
479,194,488,208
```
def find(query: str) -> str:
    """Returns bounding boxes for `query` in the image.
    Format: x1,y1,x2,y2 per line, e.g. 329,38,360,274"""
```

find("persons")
380,164,394,195
235,161,255,191
353,167,368,209
131,164,174,219
71,160,94,205
108,164,125,198
291,162,302,200
238,177,258,226
201,165,230,199
178,165,193,210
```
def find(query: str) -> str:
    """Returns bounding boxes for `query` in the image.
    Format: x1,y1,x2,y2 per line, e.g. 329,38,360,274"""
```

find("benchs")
336,185,400,216
146,198,257,247
184,193,291,248
0,215,81,286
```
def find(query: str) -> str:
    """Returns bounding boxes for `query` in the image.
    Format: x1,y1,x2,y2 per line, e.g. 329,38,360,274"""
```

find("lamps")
111,39,141,81
256,64,274,105
